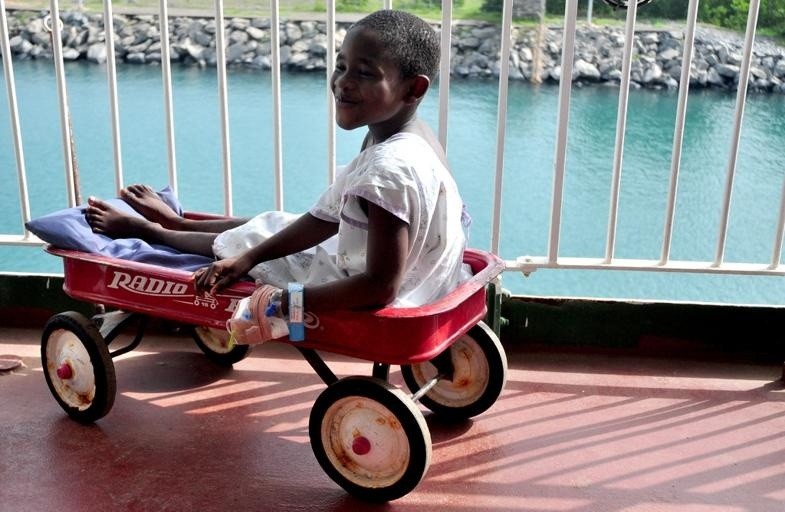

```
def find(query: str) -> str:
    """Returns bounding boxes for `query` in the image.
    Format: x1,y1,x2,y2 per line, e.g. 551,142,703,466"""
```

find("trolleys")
39,212,511,504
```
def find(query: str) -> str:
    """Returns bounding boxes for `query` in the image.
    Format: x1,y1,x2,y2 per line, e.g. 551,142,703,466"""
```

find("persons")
85,11,465,317
